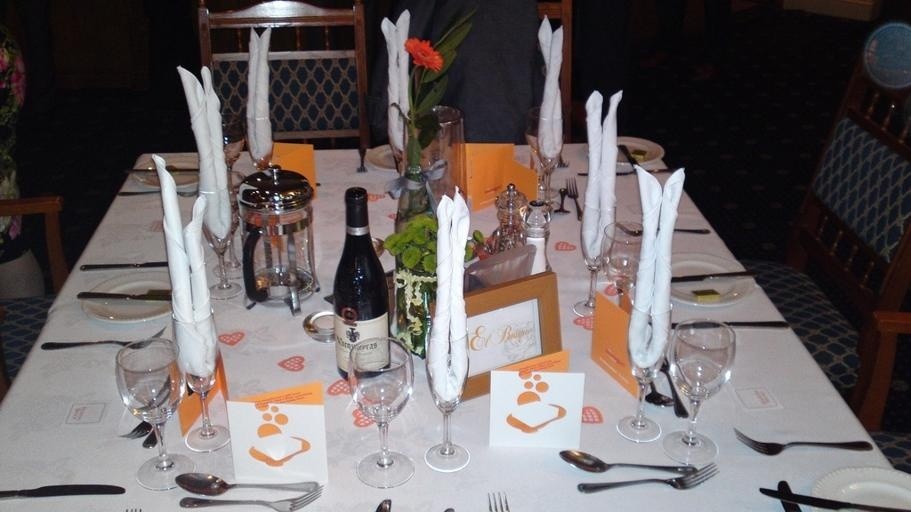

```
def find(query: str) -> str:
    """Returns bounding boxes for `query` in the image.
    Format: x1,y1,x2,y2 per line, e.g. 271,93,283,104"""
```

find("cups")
401,105,466,215
236,165,318,313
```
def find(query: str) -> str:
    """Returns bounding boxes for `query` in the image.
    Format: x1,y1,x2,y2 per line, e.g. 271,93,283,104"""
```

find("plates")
367,144,398,171
670,250,754,307
81,151,201,323
812,465,910,510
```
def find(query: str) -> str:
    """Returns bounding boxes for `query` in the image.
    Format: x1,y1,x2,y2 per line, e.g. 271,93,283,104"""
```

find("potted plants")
384,206,477,359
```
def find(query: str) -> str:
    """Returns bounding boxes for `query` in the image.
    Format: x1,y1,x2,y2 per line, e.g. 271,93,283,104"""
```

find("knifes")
0,483,126,498
672,321,791,329
759,481,910,511
670,270,758,281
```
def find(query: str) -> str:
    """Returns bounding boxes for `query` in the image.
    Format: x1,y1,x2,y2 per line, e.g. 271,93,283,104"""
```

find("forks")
577,463,720,494
488,492,509,512
40,324,167,349
732,428,875,456
179,485,325,512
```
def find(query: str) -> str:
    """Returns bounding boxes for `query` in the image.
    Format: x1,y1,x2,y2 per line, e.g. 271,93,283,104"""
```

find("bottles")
487,184,553,277
334,187,390,382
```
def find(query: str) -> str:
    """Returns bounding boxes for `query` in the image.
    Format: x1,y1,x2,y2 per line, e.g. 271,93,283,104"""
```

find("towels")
582,91,625,261
536,18,566,161
246,23,273,160
147,153,220,378
176,65,235,242
380,8,412,152
426,187,471,399
628,163,687,369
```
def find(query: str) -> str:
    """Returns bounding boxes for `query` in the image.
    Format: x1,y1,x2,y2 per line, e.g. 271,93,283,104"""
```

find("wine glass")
349,322,469,488
198,113,273,301
114,310,231,492
526,105,737,469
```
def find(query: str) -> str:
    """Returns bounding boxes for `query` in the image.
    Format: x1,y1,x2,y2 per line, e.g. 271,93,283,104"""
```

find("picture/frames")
430,272,561,403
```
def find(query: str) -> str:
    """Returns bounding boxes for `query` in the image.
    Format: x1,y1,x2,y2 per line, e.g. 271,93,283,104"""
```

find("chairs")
198,0,370,151
536,0,576,144
736,16,910,410
849,312,911,476
0,194,70,405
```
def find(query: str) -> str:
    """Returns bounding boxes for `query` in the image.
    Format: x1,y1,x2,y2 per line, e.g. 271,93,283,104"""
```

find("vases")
394,167,436,271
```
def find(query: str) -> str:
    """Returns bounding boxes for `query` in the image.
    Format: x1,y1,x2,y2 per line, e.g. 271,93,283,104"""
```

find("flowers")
392,7,476,221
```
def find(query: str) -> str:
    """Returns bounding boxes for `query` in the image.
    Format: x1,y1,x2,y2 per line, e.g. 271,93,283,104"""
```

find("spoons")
560,449,698,474
174,473,319,495
